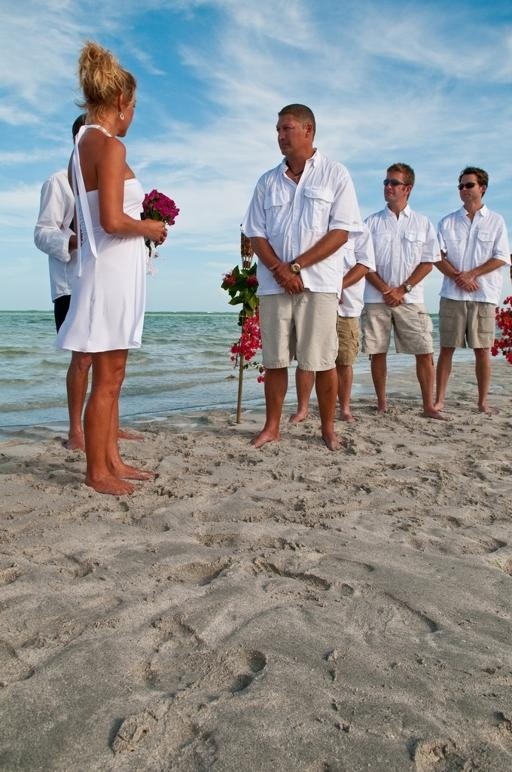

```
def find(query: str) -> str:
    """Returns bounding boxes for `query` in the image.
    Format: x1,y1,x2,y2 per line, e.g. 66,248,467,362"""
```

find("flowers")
488,294,512,366
140,189,179,254
221,263,264,383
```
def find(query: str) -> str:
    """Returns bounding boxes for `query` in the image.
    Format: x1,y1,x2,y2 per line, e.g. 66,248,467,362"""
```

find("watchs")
290,259,301,274
403,281,412,292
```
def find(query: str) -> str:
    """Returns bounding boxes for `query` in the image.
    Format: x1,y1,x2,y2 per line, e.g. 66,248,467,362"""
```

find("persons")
238,104,364,449
53,41,166,495
360,163,442,419
34,114,144,450
430,166,512,414
291,214,376,423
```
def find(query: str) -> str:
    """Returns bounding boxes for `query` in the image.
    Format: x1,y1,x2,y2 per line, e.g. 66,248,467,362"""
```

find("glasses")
458,182,476,190
383,178,407,186
